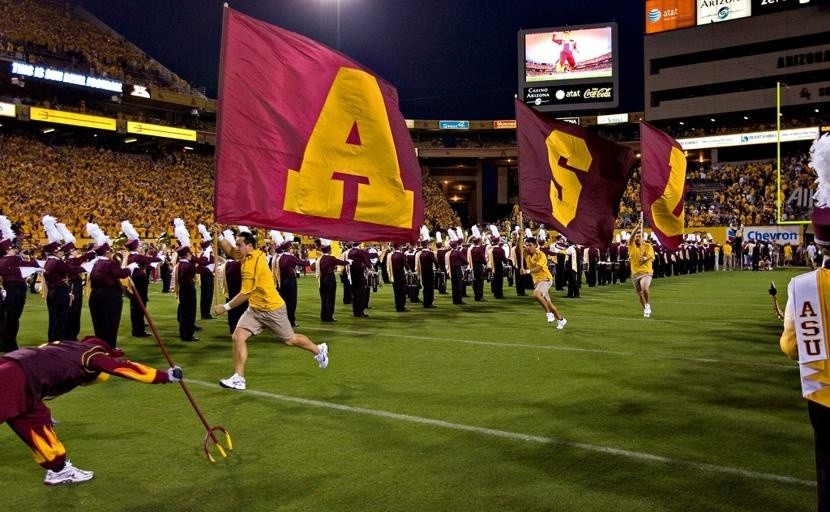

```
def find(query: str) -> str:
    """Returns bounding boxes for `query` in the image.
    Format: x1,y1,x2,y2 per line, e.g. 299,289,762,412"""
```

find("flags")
212,4,424,245
512,97,631,256
640,119,687,251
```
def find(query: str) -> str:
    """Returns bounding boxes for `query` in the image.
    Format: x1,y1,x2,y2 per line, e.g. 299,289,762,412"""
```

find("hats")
646,236,714,250
273,235,502,252
812,206,830,247
0,239,214,252
537,233,629,248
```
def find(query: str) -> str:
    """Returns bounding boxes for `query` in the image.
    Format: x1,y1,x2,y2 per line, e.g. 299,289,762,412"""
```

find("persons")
682,152,818,227
1,335,185,486
1,93,309,278
496,196,520,231
615,166,642,229
0,0,190,96
551,228,722,298
419,162,463,235
552,31,580,72
0,210,313,348
777,207,830,512
723,222,822,267
212,231,332,392
319,226,549,323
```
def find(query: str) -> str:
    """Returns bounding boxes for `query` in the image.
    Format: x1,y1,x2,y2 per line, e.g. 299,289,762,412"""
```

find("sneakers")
314,341,328,370
41,461,97,488
543,312,569,330
642,303,654,318
220,373,247,391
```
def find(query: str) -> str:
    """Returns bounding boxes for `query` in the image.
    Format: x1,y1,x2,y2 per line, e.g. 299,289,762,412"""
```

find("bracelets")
224,303,232,311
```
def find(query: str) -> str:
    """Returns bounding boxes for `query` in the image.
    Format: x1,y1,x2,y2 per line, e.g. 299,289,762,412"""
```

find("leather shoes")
556,267,735,299
284,278,535,329
130,314,219,345
